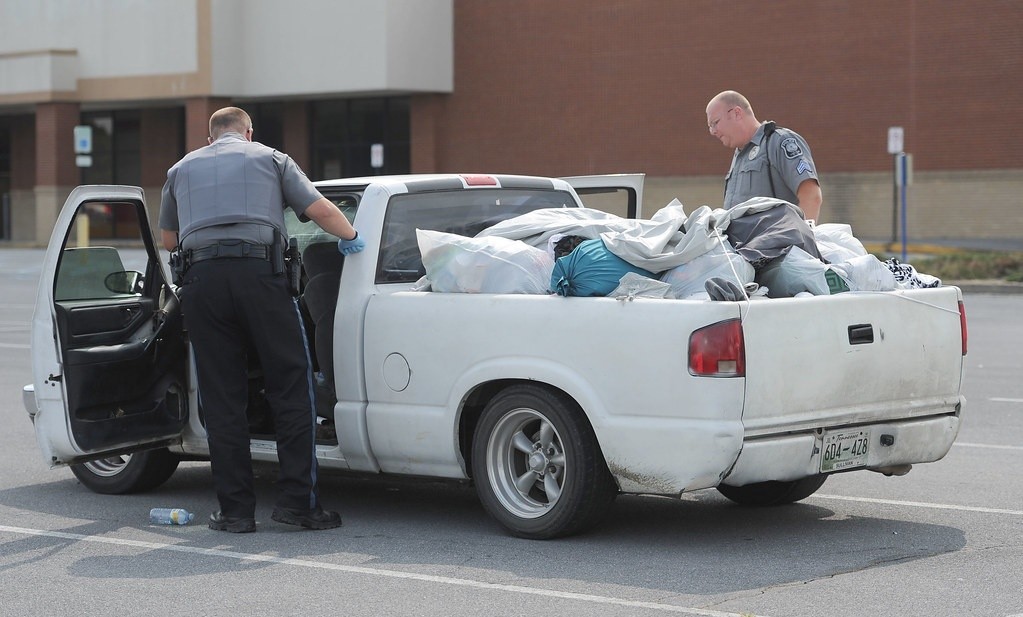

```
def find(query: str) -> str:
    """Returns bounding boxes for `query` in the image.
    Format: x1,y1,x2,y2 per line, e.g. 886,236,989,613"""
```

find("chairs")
301,241,346,424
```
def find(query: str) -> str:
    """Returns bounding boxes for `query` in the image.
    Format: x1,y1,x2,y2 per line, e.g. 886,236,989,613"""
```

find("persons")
158,107,365,533
705,90,822,226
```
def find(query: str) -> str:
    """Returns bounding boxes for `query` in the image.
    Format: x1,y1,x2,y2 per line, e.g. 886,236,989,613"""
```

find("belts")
191,244,270,264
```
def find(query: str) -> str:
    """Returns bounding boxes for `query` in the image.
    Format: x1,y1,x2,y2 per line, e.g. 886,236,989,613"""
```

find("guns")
287,237,303,297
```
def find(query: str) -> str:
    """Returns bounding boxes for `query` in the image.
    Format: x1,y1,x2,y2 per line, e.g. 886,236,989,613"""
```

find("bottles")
150,508,195,525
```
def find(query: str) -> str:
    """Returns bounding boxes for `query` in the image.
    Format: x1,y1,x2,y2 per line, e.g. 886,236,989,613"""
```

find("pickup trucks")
22,174,967,541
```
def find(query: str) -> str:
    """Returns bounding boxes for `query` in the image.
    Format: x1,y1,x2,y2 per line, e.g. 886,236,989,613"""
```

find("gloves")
337,231,364,256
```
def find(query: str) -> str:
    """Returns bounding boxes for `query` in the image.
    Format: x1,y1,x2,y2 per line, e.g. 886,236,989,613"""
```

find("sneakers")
272,505,342,530
210,509,256,532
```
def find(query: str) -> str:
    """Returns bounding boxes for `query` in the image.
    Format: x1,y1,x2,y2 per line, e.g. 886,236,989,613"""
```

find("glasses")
708,108,734,131
